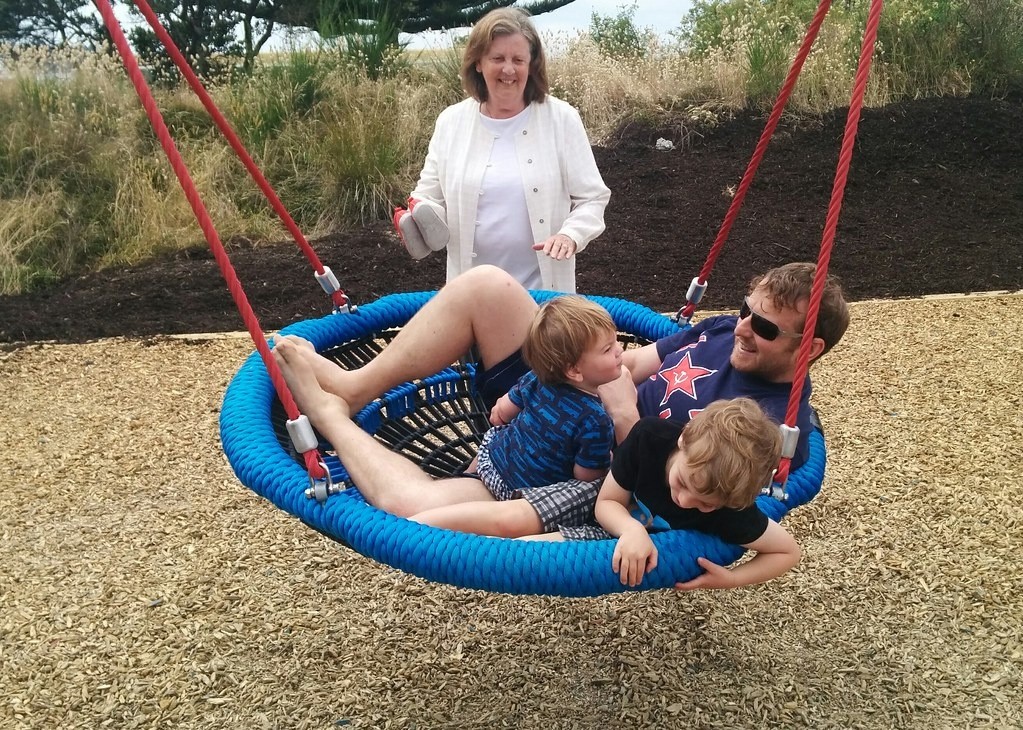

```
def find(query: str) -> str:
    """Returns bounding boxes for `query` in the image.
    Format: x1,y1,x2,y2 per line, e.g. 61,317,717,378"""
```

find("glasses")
740,295,804,341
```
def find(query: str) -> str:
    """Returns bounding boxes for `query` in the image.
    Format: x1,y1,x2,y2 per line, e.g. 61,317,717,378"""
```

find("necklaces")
578,388,597,397
485,100,493,118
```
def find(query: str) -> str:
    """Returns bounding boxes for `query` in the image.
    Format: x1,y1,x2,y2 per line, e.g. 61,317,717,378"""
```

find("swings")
88,0,891,603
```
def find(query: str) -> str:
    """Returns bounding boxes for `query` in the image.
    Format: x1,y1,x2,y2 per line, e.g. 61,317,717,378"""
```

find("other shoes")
407,197,450,251
395,207,433,261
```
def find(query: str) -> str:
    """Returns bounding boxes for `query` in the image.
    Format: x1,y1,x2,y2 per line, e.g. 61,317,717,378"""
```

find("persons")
270,261,850,588
405,8,611,294
